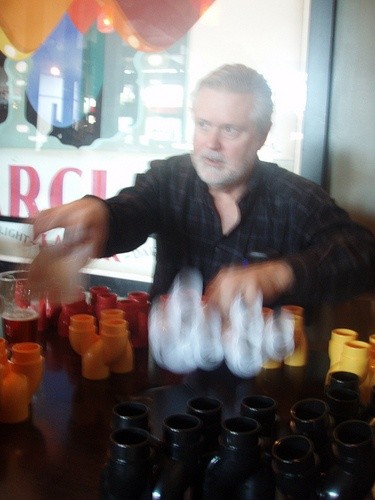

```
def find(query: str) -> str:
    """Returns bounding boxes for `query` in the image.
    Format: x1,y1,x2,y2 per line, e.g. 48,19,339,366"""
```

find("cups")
0,271,153,423
108,305,375,500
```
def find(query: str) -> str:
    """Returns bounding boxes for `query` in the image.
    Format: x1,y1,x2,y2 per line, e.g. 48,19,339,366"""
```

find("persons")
33,64,375,325
